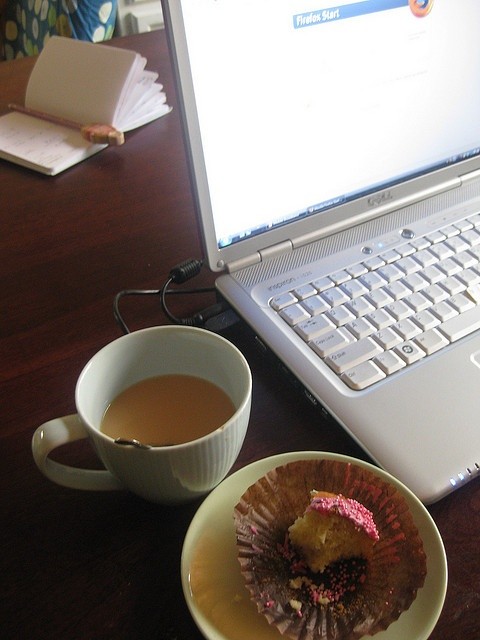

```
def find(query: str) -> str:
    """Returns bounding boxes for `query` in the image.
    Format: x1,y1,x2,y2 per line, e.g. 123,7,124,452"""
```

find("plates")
177,449,449,638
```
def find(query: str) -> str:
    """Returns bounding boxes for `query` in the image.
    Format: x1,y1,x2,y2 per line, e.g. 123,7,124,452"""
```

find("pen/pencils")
8,103,125,146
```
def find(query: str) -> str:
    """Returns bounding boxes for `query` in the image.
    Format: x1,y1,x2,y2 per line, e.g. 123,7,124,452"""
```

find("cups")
31,324,252,505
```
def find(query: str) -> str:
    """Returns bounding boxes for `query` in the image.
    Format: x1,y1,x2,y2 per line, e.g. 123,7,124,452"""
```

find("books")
1,35,174,176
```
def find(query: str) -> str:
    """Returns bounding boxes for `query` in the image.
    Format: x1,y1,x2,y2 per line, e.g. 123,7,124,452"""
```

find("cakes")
288,489,379,572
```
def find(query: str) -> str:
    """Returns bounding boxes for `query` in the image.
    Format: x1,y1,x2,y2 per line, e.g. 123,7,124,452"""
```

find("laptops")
161,0,480,505
